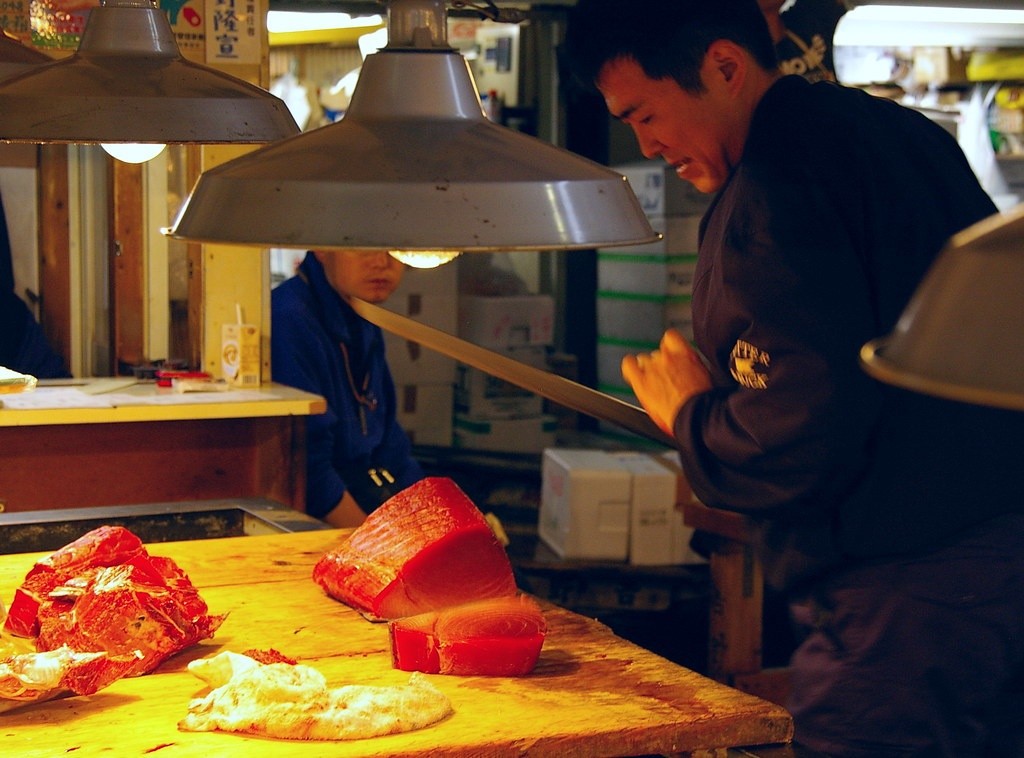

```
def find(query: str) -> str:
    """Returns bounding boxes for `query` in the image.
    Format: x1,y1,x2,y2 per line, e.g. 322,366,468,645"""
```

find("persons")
271,250,532,593
565,0,1024,758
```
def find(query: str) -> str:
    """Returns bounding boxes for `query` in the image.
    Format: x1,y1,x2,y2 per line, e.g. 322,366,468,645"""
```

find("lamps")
0,0,304,164
162,0,662,269
858,203,1024,411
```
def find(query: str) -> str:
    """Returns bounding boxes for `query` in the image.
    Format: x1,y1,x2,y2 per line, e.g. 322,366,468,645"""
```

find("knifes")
344,293,678,451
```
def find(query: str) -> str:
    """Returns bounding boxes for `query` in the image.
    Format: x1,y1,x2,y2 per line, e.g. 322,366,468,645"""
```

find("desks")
0,527,795,758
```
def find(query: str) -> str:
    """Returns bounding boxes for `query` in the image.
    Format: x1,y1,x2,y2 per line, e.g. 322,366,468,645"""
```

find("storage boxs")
379,160,707,564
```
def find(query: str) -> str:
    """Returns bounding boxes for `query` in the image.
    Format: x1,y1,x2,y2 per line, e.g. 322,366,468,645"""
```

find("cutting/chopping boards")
0,528,794,758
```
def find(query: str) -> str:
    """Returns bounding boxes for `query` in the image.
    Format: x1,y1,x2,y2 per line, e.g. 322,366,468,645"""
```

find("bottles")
480,89,500,122
